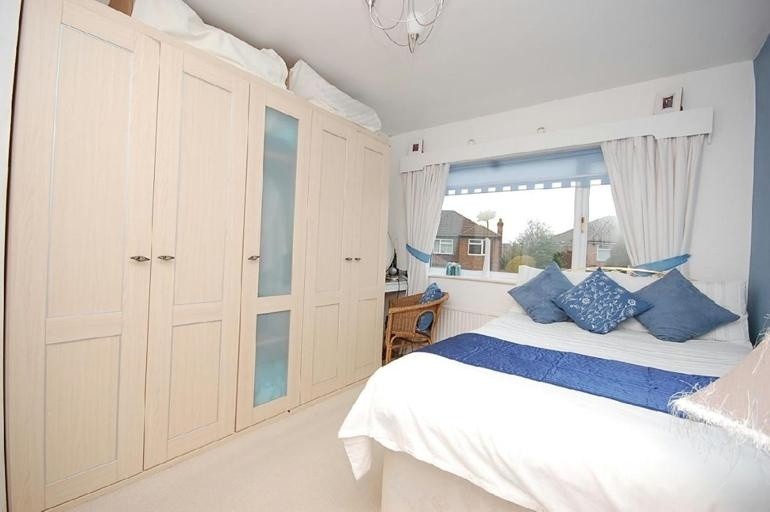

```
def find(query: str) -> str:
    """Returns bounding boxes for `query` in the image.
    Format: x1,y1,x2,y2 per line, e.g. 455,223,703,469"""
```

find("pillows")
631,268,741,343
503,263,575,325
551,264,656,336
415,282,443,331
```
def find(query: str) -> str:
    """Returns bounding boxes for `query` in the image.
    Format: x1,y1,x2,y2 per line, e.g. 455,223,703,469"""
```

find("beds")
336,263,770,512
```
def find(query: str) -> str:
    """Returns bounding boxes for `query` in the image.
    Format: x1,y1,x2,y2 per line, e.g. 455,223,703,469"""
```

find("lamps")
361,0,443,57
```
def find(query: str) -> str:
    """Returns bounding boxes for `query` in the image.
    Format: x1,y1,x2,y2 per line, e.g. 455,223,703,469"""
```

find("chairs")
384,289,449,364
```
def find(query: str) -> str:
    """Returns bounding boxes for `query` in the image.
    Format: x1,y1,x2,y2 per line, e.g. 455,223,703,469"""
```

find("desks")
384,278,408,304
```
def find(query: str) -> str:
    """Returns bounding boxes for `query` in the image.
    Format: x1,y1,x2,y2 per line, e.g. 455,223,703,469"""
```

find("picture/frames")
653,86,682,116
408,139,424,155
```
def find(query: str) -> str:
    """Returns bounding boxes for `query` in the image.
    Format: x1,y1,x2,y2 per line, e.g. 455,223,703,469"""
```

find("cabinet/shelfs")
307,105,389,406
233,71,306,432
2,0,241,512
